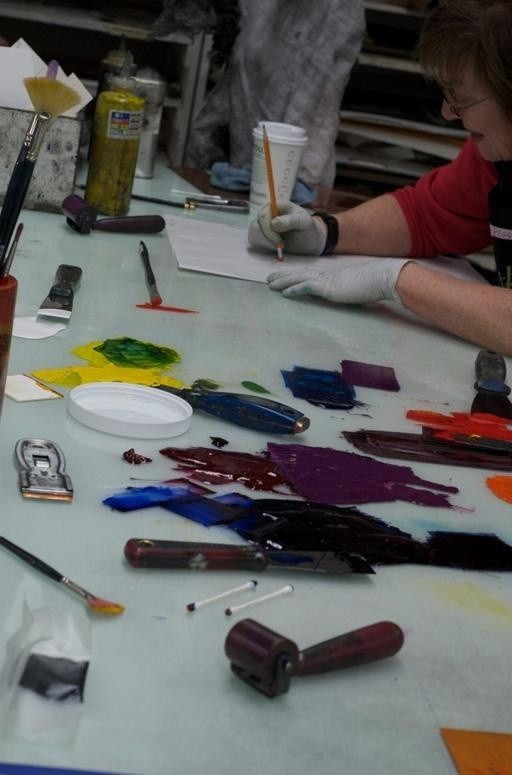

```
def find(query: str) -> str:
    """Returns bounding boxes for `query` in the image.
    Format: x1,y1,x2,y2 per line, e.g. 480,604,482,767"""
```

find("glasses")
441,91,493,116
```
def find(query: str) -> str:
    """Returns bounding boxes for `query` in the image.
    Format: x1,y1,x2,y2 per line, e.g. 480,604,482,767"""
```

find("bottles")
84,91,145,218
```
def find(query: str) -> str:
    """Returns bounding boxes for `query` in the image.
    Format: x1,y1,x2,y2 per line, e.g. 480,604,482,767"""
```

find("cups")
250,121,307,219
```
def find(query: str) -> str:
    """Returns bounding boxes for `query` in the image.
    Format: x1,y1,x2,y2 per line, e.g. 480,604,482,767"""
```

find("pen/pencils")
74,183,195,211
262,125,284,260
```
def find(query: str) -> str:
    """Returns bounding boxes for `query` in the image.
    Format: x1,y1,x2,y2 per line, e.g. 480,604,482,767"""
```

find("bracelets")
311,211,340,256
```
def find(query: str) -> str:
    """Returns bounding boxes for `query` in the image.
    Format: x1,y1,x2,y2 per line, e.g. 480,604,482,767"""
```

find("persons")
247,0,512,357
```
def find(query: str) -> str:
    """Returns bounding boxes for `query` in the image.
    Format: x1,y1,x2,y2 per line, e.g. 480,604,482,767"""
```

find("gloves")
247,199,326,256
266,257,417,305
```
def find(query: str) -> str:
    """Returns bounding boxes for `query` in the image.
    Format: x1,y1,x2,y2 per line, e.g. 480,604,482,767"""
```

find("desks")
1,194,512,775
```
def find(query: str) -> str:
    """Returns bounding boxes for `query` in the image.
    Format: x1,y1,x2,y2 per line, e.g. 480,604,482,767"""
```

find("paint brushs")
3,224,26,275
139,240,162,305
1,535,125,616
0,59,59,216
0,77,80,271
